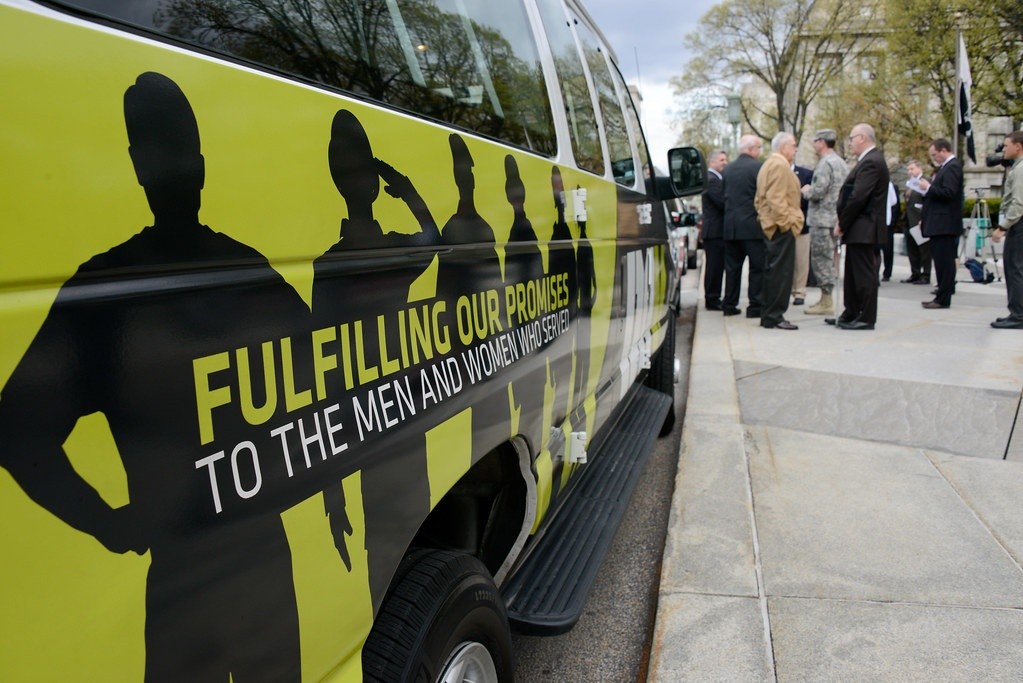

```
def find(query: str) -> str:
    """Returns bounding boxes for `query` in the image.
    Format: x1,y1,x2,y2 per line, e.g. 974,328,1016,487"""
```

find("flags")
957,33,977,166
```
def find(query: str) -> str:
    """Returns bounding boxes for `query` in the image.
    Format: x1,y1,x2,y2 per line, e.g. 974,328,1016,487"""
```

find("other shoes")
705,301,723,310
900,277,915,283
725,306,741,316
914,279,930,284
881,277,890,281
793,298,804,305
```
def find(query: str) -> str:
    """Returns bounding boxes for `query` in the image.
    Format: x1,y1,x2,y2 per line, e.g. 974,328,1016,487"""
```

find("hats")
811,128,836,141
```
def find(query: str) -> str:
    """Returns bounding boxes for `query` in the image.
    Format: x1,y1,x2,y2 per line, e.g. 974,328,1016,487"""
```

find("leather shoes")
991,315,1023,330
840,319,874,330
773,319,797,330
922,296,947,309
825,314,855,325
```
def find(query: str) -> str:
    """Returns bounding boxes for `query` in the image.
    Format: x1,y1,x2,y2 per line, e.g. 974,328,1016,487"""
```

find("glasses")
849,135,864,141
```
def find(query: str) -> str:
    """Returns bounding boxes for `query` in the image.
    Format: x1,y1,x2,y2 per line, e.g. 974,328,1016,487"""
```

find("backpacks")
965,257,995,284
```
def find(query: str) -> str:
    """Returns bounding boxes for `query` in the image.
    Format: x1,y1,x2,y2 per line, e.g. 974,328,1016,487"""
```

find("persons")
801,128,850,315
990,130,1023,330
753,131,805,329
700,134,819,317
919,138,964,308
825,123,890,329
901,159,931,285
881,180,901,282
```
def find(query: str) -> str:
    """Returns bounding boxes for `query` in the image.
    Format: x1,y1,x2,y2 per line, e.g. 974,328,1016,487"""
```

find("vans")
651,166,703,274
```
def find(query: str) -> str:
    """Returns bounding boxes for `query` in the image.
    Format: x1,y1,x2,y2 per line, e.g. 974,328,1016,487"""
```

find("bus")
0,0,712,681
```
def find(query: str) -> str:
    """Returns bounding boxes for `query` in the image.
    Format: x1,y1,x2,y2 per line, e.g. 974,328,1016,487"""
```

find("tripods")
959,197,1002,282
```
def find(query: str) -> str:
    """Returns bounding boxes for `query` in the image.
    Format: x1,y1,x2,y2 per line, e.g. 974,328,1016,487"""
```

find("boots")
803,293,833,314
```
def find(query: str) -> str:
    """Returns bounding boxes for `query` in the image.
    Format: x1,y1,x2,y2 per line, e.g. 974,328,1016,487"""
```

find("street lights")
726,94,744,164
